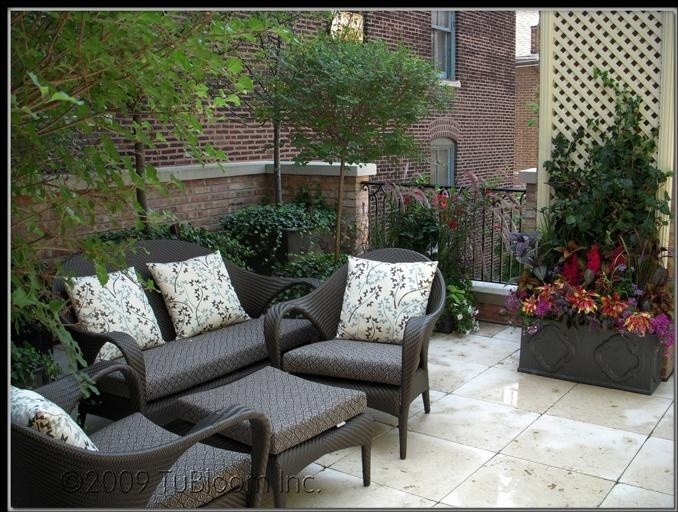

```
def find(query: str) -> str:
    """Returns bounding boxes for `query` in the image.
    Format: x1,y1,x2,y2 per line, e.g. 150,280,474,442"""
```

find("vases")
436,313,455,335
515,314,664,395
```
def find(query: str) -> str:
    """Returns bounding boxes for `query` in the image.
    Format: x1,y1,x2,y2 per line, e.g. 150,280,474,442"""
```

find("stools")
174,364,373,509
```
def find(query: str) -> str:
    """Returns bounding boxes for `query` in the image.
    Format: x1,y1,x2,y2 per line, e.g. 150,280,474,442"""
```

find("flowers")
495,229,674,353
402,181,503,336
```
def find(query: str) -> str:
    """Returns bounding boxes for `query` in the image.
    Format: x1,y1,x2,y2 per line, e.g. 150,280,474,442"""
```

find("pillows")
144,250,252,342
59,266,167,367
10,385,99,453
333,254,438,346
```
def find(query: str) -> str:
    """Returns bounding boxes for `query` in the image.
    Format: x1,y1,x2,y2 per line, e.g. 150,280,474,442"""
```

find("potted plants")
215,201,353,261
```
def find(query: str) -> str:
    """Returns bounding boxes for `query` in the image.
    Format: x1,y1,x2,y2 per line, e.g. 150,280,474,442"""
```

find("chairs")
263,247,446,460
52,239,322,424
6,363,270,512
